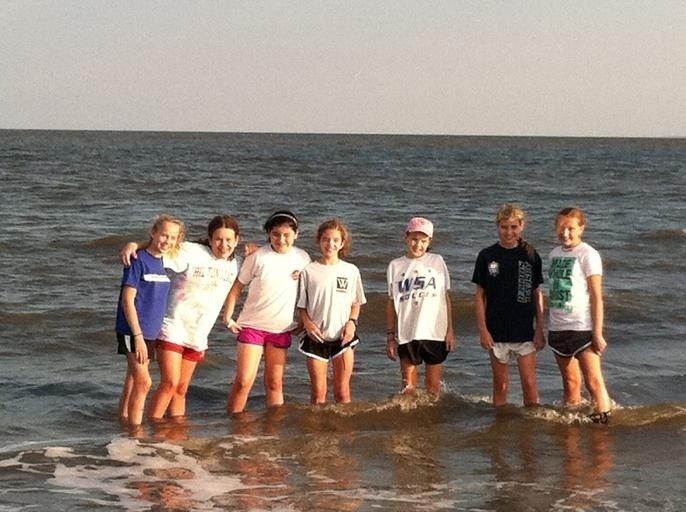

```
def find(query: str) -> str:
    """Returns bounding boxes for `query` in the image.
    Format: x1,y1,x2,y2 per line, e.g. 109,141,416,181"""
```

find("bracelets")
387,328,395,335
226,319,233,328
133,332,143,336
349,318,359,330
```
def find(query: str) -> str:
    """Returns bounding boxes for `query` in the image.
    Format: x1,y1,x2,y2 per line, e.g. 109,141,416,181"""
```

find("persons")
296,218,368,405
115,213,185,425
119,213,257,420
223,209,312,414
546,207,610,413
385,216,455,395
471,202,546,406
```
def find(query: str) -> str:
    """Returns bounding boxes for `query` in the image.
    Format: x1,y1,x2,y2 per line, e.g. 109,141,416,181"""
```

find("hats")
405,217,433,238
